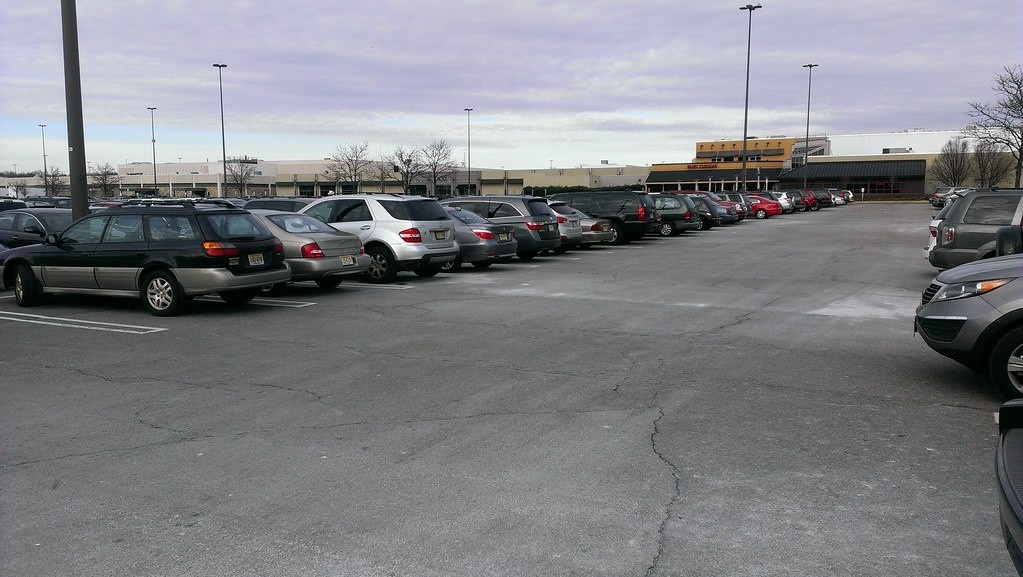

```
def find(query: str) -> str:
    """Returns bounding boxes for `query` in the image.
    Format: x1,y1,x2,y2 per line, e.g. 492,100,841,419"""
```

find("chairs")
124,232,141,240
25,218,37,228
160,227,181,239
272,217,285,232
0,219,13,229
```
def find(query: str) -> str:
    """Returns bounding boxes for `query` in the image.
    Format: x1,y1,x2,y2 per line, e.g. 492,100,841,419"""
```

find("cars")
912,250,1023,400
0,195,259,254
227,208,371,298
993,397,1023,577
545,186,854,256
443,206,519,272
0,196,293,318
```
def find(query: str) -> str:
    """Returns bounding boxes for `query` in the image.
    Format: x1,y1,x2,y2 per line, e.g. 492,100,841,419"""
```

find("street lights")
212,63,230,199
147,107,157,195
464,108,474,196
38,125,48,196
802,64,819,188
738,3,762,194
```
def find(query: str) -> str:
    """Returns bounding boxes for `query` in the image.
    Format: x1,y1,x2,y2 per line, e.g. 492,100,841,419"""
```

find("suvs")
283,191,462,284
923,185,1023,268
436,193,562,261
